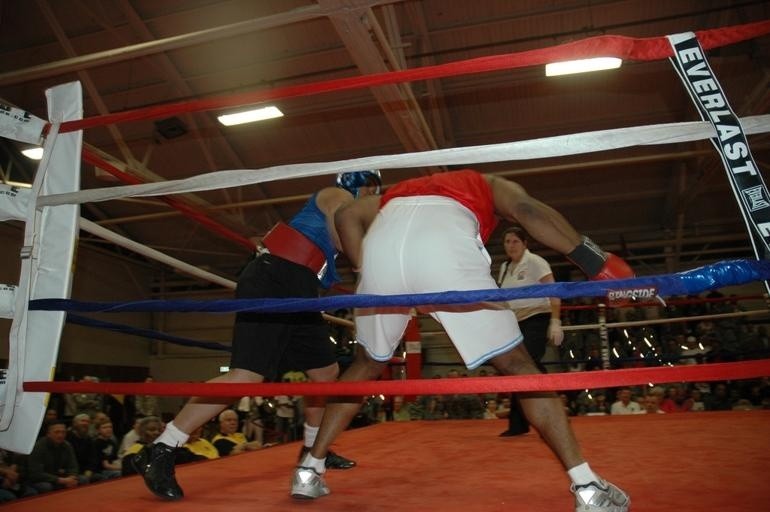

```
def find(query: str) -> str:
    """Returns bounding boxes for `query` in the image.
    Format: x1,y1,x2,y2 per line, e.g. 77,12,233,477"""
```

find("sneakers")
568,478,631,512
297,445,357,470
289,466,331,499
497,425,530,438
129,441,184,499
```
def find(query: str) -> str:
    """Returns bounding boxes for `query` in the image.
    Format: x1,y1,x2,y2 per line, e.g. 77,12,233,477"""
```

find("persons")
128,167,386,503
0,285,769,506
285,168,643,512
493,225,567,438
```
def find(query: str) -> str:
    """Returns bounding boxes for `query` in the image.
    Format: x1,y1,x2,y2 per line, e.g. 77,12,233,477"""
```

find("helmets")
336,167,383,200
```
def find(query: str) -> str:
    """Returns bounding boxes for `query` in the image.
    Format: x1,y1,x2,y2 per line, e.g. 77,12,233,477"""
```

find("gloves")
564,234,638,309
547,318,565,346
